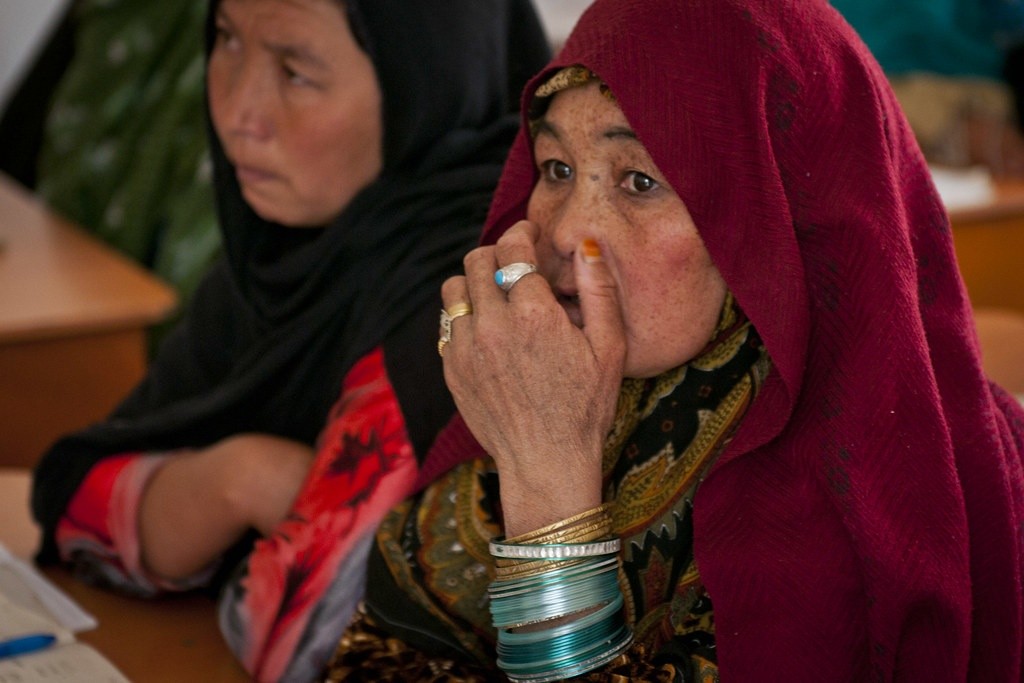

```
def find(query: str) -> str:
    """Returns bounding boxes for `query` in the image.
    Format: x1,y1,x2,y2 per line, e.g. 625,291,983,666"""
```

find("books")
0,542,134,683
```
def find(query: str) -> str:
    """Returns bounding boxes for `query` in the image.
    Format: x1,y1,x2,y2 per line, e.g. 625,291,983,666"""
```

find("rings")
438,336,449,356
440,302,472,341
495,262,539,291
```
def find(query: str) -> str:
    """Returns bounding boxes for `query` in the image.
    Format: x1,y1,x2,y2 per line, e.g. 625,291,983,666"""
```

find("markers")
0,635,54,660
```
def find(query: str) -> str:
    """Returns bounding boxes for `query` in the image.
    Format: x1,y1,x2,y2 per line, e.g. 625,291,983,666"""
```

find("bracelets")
490,499,635,683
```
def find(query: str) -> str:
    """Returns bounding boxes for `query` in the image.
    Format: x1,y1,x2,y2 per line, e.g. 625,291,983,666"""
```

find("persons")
320,0,1023,683
28,0,557,683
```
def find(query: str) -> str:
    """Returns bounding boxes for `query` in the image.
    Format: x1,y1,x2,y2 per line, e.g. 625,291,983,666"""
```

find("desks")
0,179,185,464
0,469,254,683
